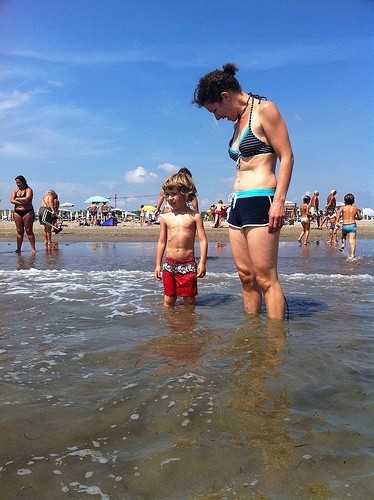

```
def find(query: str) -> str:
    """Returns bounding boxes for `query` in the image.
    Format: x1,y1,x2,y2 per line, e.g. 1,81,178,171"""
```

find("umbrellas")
142,206,158,212
60,203,74,208
326,201,345,207
85,195,110,204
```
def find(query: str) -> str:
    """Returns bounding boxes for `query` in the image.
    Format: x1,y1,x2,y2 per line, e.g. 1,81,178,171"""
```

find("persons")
155,174,208,308
11,168,361,259
193,64,296,319
